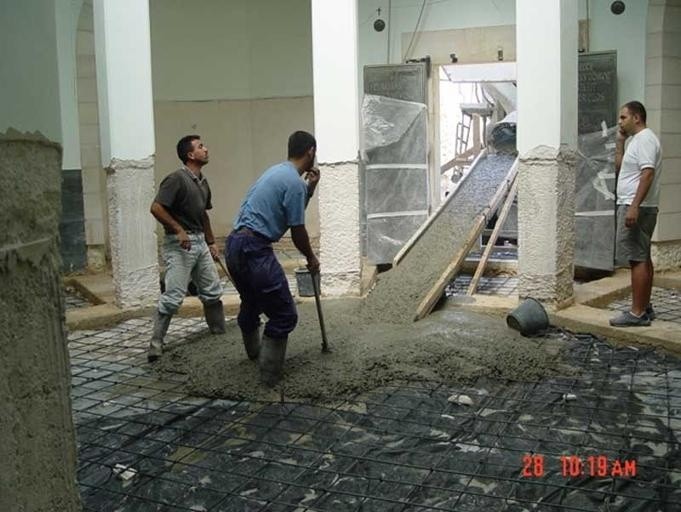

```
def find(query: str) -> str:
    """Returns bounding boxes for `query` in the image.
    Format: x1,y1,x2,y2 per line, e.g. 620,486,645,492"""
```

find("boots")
260,334,287,384
243,330,261,359
203,301,226,336
148,312,171,361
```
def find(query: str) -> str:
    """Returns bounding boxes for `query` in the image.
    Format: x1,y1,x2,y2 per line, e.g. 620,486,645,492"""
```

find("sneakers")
609,311,650,326
622,306,656,320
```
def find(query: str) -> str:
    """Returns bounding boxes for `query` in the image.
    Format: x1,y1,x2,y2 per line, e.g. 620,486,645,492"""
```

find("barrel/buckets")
295,263,322,298
506,297,550,336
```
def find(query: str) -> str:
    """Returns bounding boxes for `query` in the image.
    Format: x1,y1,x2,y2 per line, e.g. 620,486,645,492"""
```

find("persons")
147,134,227,362
224,130,321,387
608,100,664,327
